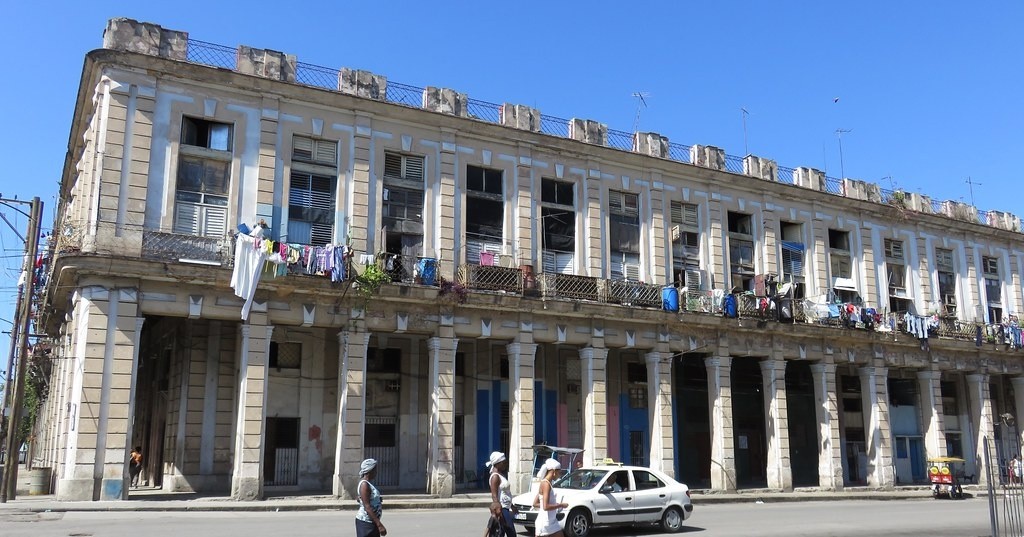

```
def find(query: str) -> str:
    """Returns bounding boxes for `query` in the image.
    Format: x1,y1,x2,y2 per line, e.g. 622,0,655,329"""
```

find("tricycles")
926,457,965,499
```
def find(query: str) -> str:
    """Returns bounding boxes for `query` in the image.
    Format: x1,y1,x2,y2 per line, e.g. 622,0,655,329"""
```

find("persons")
598,475,621,492
355,459,387,537
531,458,569,537
1009,455,1024,498
484,451,518,537
129,446,143,489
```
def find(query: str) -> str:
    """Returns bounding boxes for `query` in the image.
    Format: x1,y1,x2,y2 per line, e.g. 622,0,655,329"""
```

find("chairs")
616,477,628,491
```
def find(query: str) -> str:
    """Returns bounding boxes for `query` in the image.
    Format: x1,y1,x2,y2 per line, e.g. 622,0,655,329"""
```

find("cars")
507,458,694,537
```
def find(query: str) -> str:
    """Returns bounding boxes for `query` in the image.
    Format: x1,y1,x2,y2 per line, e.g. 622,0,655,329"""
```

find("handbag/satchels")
486,515,505,537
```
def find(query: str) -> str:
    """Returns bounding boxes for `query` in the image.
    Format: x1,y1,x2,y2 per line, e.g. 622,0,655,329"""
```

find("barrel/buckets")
724,294,736,318
780,299,791,318
29,467,52,495
662,287,679,311
519,265,534,289
421,257,436,285
992,324,1004,344
